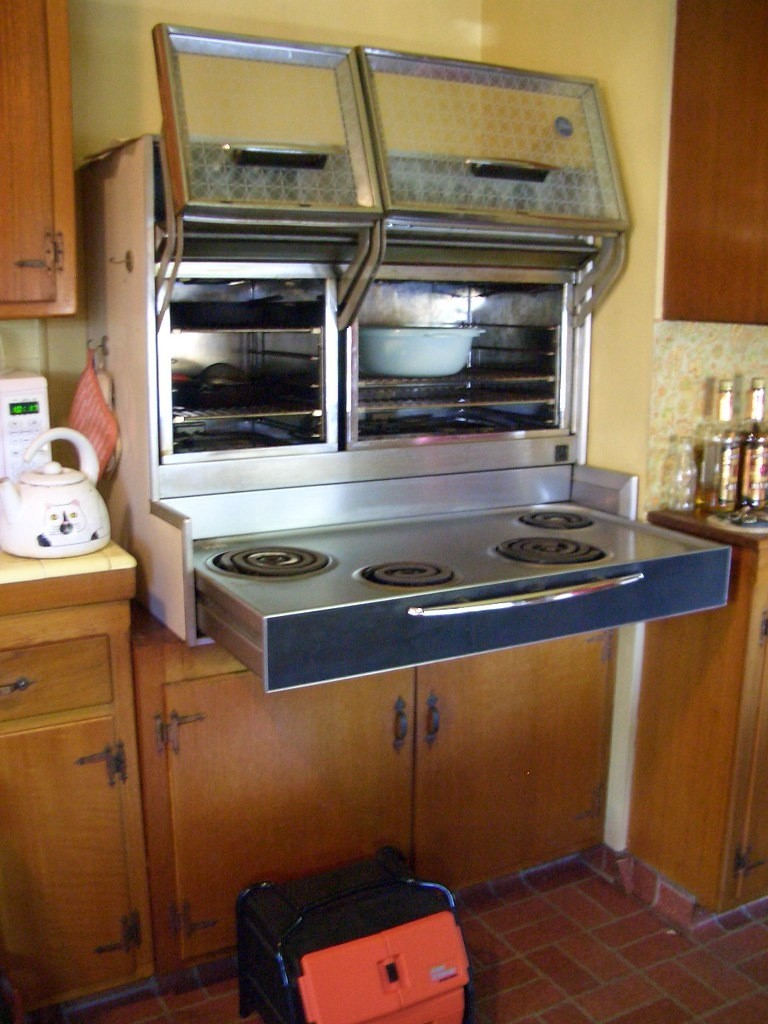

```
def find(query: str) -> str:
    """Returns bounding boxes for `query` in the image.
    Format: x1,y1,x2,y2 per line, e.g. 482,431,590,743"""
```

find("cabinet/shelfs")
1,0,80,318
3,547,156,1016
132,601,618,974
627,507,768,917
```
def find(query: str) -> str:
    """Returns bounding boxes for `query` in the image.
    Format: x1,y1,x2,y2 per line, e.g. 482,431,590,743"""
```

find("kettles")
0,427,111,559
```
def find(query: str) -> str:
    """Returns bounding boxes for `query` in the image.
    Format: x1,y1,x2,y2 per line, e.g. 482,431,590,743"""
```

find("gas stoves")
186,467,732,694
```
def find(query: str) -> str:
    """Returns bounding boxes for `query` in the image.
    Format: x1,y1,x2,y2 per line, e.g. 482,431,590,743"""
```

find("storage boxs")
233,844,476,1024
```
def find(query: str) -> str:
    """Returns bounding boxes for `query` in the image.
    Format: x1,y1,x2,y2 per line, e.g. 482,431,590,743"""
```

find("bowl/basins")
359,328,479,377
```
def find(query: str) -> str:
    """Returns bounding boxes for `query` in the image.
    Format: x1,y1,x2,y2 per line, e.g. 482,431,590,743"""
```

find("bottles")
701,380,741,513
668,437,697,512
738,378,768,520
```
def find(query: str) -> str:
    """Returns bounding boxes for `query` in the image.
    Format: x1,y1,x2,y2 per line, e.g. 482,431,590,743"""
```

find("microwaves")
0,364,53,480
